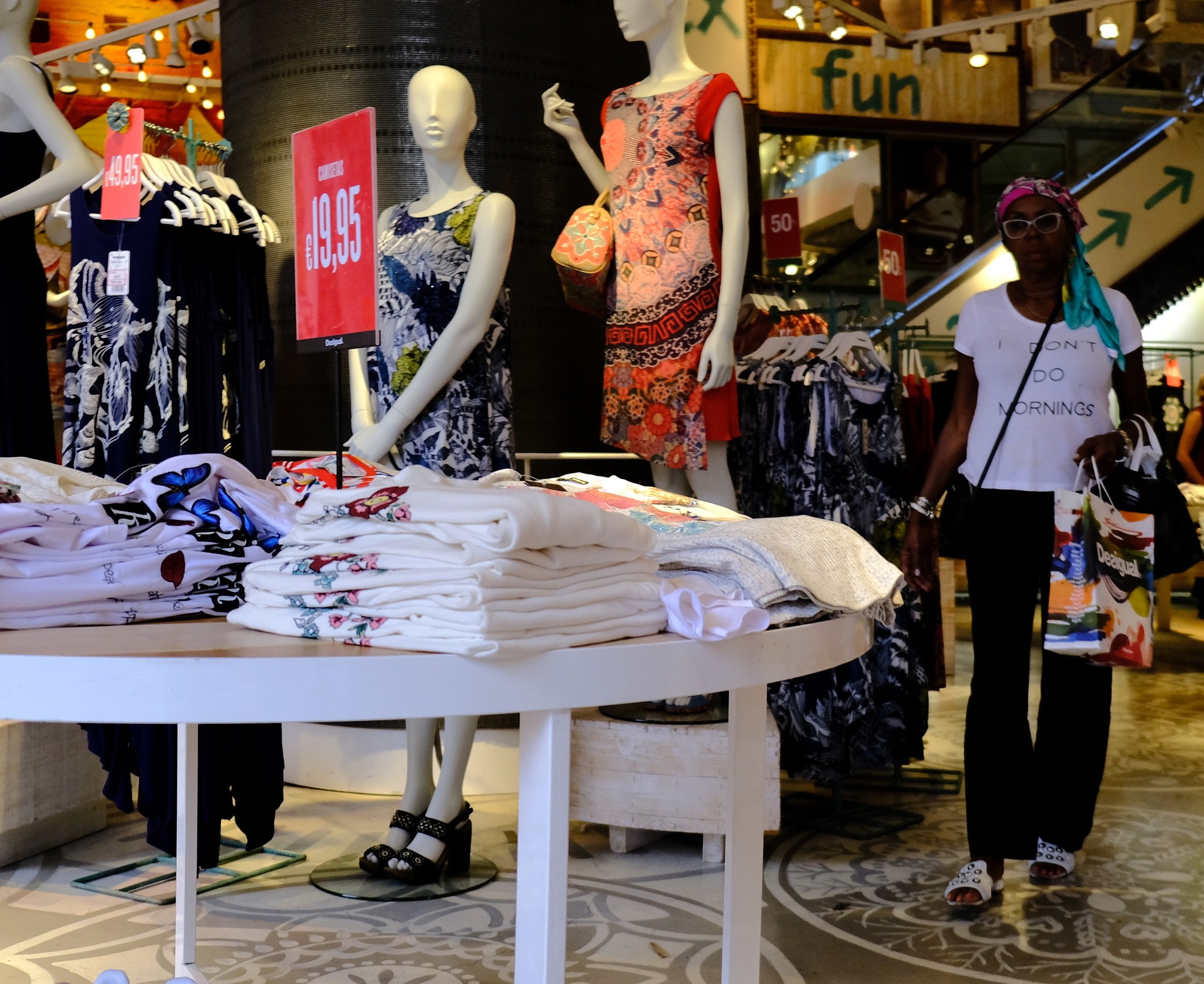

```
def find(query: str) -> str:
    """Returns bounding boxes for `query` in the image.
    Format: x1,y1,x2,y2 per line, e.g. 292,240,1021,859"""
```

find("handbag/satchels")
935,473,972,561
1043,454,1158,669
550,188,615,317
1081,409,1203,580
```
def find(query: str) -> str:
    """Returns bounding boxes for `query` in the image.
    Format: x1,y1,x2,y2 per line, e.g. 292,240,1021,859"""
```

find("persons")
343,65,515,887
540,0,749,708
0,0,96,462
899,175,1155,915
1176,378,1204,484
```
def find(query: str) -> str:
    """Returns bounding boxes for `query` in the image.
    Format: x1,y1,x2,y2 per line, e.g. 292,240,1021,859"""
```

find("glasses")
1002,212,1066,241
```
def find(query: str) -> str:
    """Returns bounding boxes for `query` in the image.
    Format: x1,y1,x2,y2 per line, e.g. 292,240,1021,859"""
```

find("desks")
0,616,874,984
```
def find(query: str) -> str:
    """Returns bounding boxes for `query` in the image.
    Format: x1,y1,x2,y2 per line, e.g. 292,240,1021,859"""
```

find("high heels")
359,797,473,880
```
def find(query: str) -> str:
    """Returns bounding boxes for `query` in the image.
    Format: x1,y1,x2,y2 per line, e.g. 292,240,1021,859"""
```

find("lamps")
58,13,214,95
1027,17,1055,47
872,31,897,60
819,6,848,41
968,34,1007,68
1097,9,1119,39
772,0,813,32
911,40,941,67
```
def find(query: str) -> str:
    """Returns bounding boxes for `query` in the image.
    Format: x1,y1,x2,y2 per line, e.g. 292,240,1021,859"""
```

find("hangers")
52,123,282,247
1146,366,1191,389
734,303,958,399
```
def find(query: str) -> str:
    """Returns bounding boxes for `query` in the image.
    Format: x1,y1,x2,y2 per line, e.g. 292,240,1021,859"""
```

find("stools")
570,706,781,864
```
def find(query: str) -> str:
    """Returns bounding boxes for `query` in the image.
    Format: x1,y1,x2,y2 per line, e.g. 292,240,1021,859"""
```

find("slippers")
942,858,1005,907
1030,836,1075,879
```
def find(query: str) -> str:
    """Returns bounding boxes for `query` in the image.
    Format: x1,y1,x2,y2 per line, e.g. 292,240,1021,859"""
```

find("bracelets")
1110,427,1133,463
909,495,938,520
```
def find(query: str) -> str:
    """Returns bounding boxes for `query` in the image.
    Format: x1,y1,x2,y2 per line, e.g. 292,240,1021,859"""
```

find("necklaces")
1026,306,1049,319
